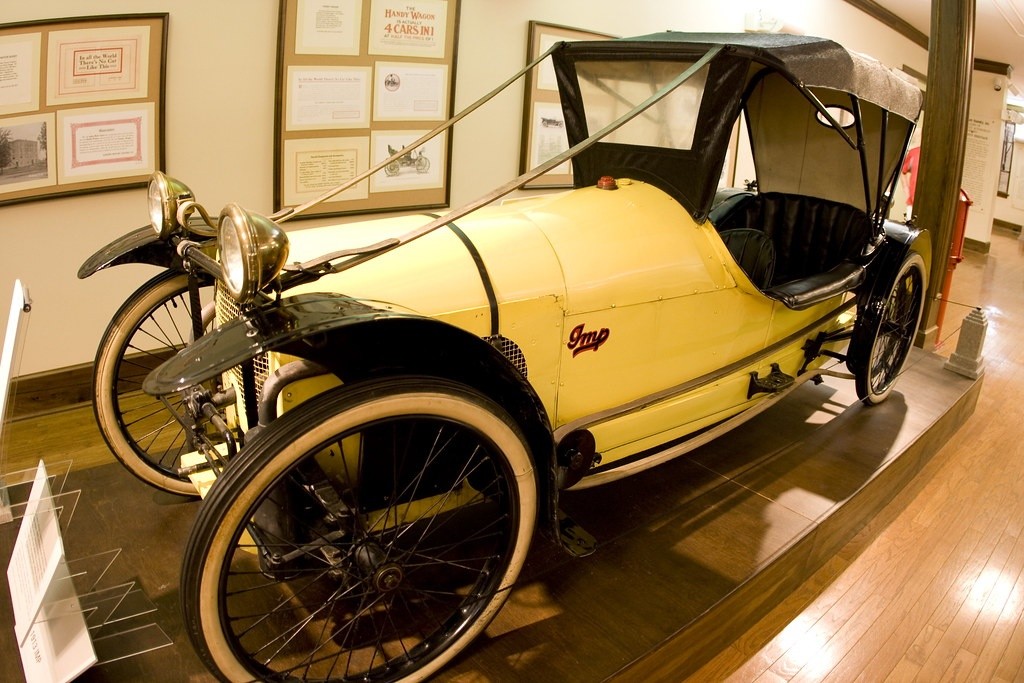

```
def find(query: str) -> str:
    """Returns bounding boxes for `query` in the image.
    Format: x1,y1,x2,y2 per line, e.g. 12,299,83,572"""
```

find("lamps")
993,76,1002,91
1006,81,1020,97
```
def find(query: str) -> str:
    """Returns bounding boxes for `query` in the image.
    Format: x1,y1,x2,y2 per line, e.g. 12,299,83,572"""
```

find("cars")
75,27,934,683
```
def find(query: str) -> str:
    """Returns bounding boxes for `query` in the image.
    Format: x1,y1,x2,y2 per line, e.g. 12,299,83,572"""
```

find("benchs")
722,191,872,281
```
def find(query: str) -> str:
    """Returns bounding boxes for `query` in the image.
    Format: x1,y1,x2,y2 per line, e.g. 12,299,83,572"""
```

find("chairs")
711,230,777,301
